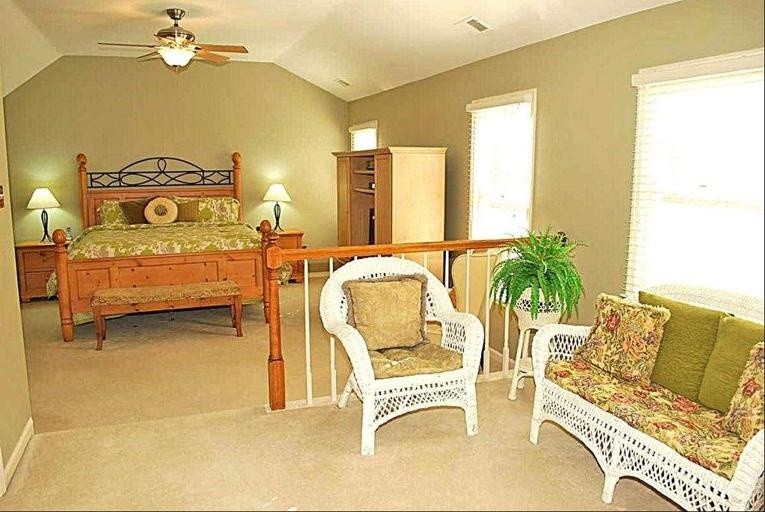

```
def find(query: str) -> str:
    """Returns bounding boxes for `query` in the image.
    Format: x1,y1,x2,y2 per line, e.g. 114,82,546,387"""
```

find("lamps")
261,182,294,231
26,186,65,243
156,48,199,71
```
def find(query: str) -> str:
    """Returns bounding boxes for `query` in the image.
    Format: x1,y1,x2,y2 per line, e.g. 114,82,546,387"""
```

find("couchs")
525,290,764,511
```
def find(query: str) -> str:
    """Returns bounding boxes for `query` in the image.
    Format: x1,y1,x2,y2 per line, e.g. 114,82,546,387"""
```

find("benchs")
86,279,244,353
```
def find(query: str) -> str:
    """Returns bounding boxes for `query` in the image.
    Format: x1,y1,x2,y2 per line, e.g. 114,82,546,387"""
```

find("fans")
98,8,246,74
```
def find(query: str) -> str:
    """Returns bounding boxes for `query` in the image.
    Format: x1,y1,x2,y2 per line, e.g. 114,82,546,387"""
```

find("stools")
504,320,563,403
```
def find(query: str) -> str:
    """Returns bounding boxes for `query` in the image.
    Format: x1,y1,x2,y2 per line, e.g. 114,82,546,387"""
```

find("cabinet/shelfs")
330,144,448,285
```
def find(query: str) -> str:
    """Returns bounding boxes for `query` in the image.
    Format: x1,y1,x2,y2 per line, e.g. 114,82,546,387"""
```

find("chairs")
315,253,487,461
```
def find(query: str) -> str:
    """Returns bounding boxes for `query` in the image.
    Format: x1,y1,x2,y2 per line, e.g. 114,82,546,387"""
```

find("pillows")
96,198,130,229
177,198,200,223
143,197,179,225
720,341,765,442
121,195,158,225
578,291,671,388
172,193,240,223
340,271,432,356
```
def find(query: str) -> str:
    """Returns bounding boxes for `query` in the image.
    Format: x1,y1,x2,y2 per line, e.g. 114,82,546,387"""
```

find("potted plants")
486,223,591,325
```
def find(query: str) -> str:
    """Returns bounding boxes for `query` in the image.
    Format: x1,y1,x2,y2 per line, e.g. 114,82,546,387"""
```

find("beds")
43,148,296,344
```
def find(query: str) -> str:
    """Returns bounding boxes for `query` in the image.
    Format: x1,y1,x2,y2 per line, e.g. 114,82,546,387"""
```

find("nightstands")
13,241,60,304
263,227,307,283
